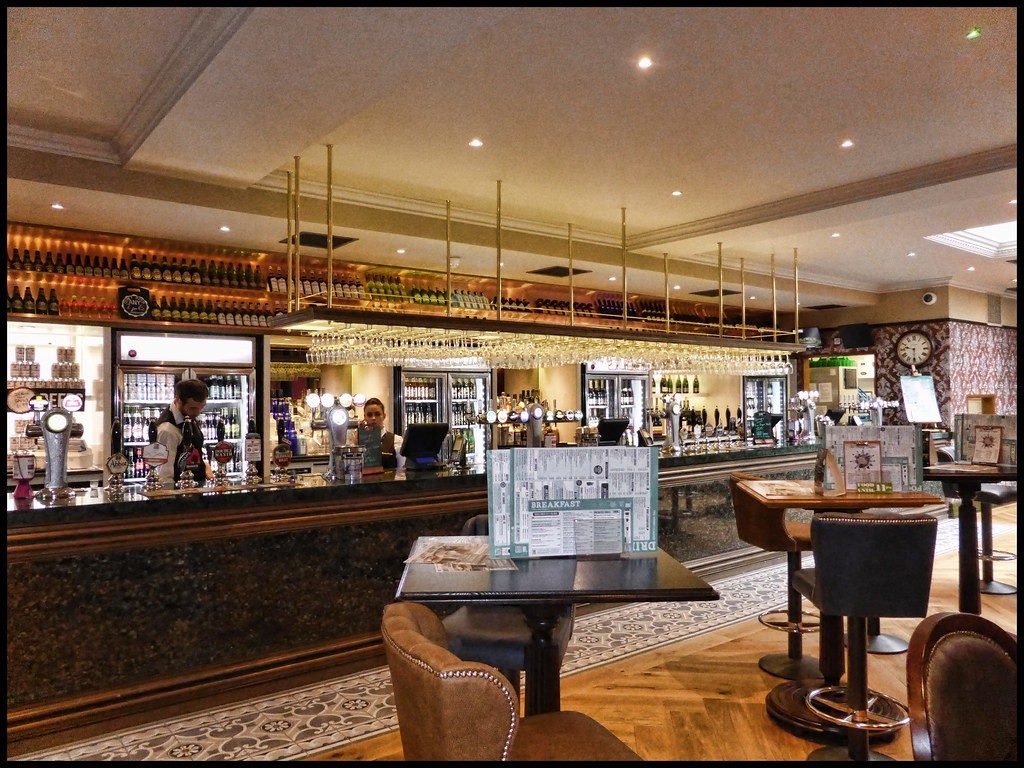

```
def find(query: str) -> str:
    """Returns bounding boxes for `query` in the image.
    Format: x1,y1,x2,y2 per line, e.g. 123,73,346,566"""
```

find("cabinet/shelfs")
744,376,787,444
401,371,492,463
585,375,649,443
117,366,249,486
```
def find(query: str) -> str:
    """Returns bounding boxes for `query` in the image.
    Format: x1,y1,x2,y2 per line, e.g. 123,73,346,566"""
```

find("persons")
357,399,407,469
151,379,210,484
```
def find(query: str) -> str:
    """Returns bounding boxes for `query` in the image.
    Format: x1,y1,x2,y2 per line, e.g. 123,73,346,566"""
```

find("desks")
921,464,1016,614
392,536,721,717
738,481,946,742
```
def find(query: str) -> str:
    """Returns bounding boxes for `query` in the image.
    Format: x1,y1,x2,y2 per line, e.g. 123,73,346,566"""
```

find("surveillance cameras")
923,292,937,305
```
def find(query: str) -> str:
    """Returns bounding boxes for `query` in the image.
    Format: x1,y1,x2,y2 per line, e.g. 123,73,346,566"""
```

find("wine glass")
306,332,794,376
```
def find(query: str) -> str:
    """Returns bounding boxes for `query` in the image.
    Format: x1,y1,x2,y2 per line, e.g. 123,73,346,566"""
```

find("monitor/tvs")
596,419,629,446
400,423,449,458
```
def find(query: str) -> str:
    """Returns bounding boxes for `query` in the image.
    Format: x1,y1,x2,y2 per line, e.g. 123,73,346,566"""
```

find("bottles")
122,374,240,477
514,422,560,447
588,379,662,445
660,375,700,394
497,390,558,416
405,377,476,460
679,400,693,432
270,388,306,456
3,250,732,330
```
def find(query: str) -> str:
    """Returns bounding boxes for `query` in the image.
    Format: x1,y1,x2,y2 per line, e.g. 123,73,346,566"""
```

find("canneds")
7,345,84,389
122,373,175,401
7,419,45,480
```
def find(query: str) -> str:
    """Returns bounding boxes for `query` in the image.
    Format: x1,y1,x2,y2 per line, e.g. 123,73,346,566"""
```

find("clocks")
894,330,932,368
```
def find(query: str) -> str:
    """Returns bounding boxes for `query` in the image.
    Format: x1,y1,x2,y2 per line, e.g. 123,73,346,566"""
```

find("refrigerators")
741,355,798,443
108,328,264,483
575,358,654,446
393,365,494,460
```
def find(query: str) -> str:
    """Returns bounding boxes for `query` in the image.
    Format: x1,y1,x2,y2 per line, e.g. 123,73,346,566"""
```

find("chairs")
937,447,1016,595
380,601,644,761
794,513,938,762
728,474,822,680
439,515,574,706
906,612,1018,762
